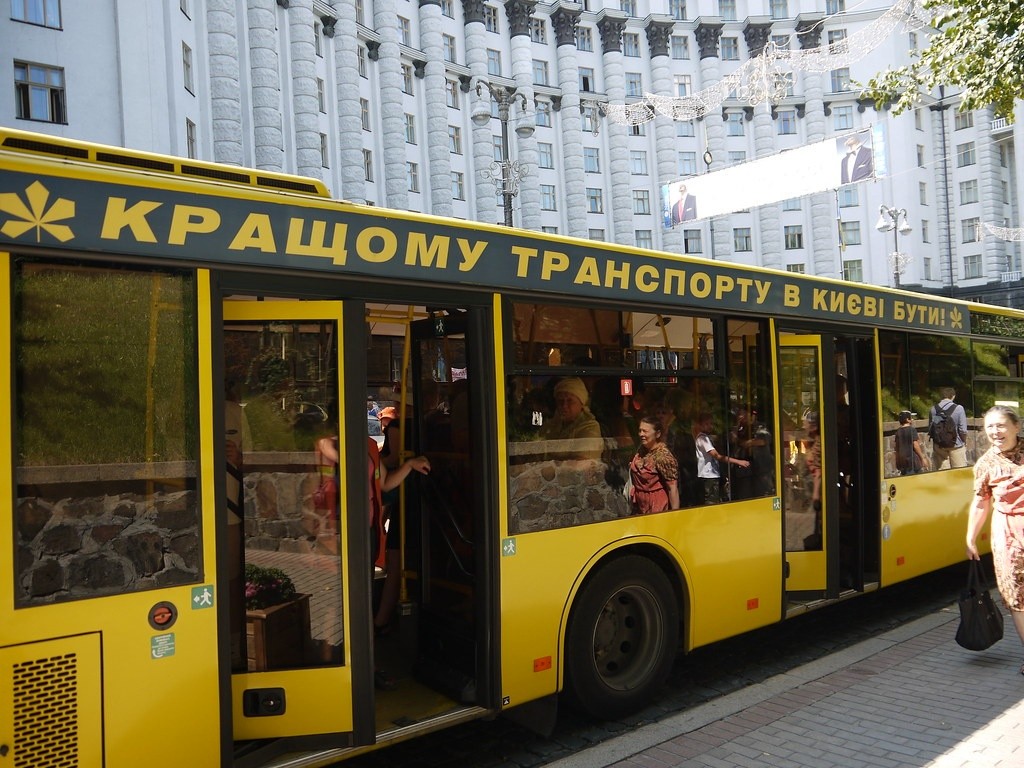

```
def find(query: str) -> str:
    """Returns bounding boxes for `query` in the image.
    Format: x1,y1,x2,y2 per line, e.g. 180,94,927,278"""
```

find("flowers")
246,564,296,610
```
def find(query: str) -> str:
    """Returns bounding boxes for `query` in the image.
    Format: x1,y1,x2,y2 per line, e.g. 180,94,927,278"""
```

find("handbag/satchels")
623,468,635,511
803,499,823,551
956,560,1006,651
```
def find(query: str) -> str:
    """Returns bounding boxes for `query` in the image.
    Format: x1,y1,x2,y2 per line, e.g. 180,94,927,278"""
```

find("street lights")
876,204,913,288
469,78,536,230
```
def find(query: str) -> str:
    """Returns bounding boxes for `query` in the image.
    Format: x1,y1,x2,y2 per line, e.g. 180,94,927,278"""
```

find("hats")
553,377,588,407
376,407,396,421
899,411,918,419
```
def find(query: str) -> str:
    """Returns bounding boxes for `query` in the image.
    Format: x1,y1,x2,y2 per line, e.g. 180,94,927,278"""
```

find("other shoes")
374,621,392,636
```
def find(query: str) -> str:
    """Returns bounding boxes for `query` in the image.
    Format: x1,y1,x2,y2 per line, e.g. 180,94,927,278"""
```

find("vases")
248,593,314,671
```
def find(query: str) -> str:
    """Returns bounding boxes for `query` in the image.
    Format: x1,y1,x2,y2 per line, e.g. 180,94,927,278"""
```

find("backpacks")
930,404,959,448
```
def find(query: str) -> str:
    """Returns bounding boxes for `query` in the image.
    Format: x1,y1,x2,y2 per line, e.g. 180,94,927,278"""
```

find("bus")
0,123,1024,768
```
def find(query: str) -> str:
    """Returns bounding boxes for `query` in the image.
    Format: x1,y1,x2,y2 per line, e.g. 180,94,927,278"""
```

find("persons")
316,397,431,662
893,387,967,475
965,407,1024,646
367,367,822,635
